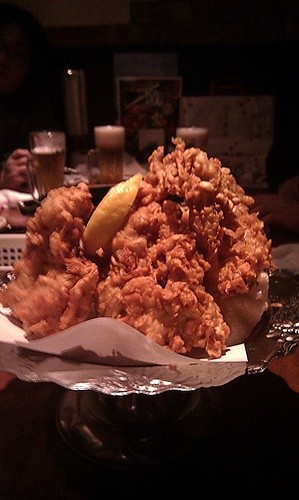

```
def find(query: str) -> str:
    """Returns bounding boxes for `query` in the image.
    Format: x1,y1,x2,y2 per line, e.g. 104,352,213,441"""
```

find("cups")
87,125,125,184
176,127,208,153
25,131,66,204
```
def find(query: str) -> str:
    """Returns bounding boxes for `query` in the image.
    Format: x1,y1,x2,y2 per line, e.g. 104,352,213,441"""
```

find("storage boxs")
114,76,183,124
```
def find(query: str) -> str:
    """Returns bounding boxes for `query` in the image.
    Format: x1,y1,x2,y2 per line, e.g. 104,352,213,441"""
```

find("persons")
247,40,299,233
0,3,74,189
